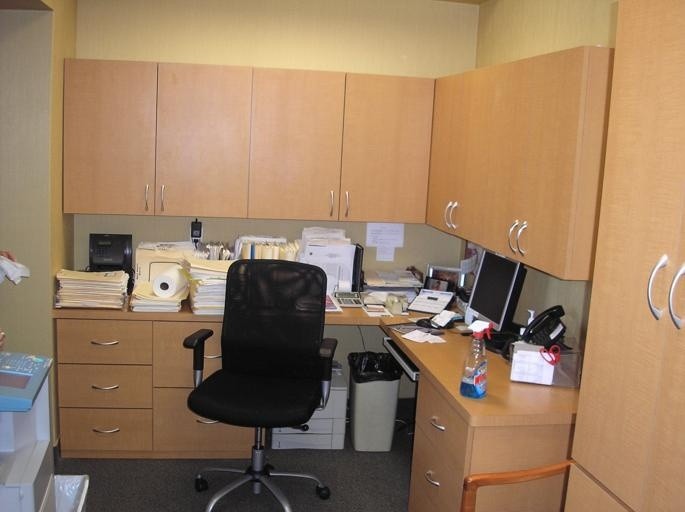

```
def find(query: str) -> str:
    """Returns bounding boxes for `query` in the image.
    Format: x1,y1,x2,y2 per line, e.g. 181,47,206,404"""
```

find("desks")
51,305,581,510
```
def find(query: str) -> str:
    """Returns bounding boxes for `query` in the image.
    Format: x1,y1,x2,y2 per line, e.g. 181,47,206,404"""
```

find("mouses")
417,318,441,330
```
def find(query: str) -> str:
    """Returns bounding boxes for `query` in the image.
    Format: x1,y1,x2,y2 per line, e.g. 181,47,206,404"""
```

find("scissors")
540,344,572,381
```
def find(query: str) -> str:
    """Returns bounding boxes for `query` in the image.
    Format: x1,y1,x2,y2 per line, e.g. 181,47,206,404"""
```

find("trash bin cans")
347,351,403,452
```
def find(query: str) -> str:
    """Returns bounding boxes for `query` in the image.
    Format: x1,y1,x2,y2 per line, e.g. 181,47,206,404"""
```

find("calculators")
335,291,364,308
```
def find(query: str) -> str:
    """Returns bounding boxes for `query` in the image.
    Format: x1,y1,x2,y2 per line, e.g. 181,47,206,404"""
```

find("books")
129,225,357,316
54,267,128,310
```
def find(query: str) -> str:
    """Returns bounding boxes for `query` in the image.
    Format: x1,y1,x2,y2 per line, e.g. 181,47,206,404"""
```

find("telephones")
521,305,573,353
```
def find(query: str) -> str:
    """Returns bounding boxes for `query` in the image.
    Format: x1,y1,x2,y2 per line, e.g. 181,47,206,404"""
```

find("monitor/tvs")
466,248,527,331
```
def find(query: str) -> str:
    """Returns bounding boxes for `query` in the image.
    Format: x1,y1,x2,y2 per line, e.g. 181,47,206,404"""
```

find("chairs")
183,257,338,512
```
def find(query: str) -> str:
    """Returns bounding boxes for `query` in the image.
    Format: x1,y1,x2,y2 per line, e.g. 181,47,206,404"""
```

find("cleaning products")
459,320,493,399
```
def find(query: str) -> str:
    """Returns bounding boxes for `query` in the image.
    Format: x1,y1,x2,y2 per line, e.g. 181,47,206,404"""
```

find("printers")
0,351,57,512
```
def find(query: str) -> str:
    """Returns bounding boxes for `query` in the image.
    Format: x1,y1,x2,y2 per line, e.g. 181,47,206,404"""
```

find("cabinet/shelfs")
56,318,266,460
563,0,685,510
427,46,612,283
64,58,253,219
405,373,575,511
252,68,427,225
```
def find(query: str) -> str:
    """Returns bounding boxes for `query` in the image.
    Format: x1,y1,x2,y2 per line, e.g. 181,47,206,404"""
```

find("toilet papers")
152,264,190,298
